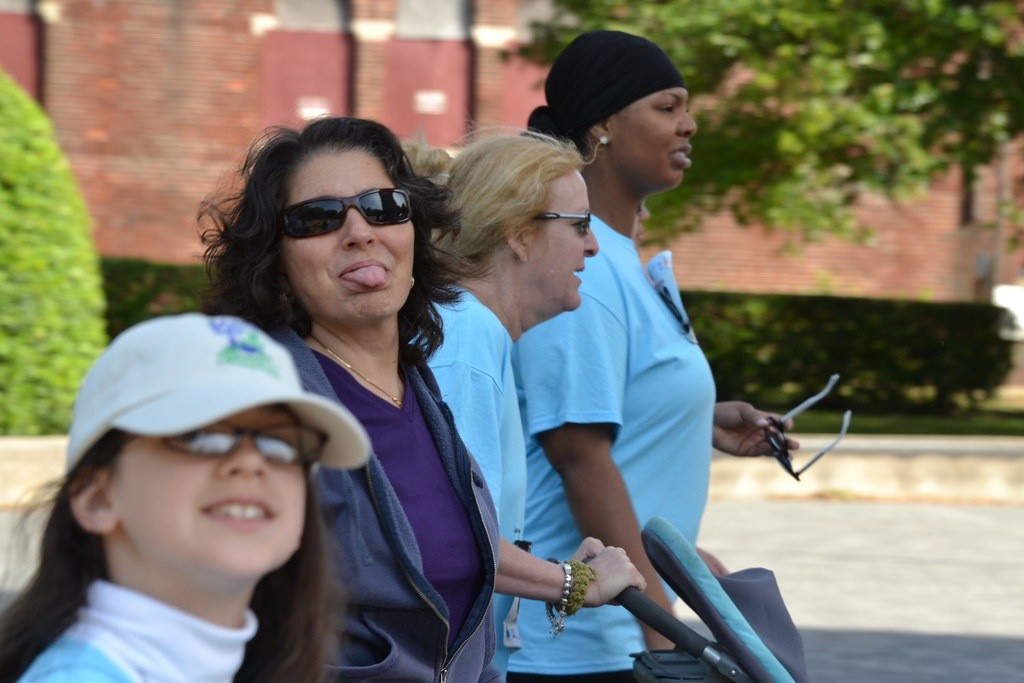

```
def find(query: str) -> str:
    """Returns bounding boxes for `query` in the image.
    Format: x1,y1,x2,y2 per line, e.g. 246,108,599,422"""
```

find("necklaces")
310,336,402,407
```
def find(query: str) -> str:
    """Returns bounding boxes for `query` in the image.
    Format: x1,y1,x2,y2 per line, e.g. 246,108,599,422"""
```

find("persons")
290,211,330,234
408,131,647,683
504,28,800,683
195,116,500,683
0,313,372,683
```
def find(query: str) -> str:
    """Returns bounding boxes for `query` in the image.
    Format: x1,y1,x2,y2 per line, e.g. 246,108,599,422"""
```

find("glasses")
764,374,853,482
166,415,329,466
530,210,591,236
277,186,412,239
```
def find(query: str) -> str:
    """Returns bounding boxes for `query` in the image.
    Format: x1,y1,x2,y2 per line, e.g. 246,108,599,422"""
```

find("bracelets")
545,557,598,639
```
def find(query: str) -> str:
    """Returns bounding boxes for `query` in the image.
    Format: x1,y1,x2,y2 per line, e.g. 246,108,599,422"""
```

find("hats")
64,312,371,478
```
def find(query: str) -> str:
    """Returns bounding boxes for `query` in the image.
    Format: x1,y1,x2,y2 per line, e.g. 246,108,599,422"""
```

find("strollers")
583,516,810,683
